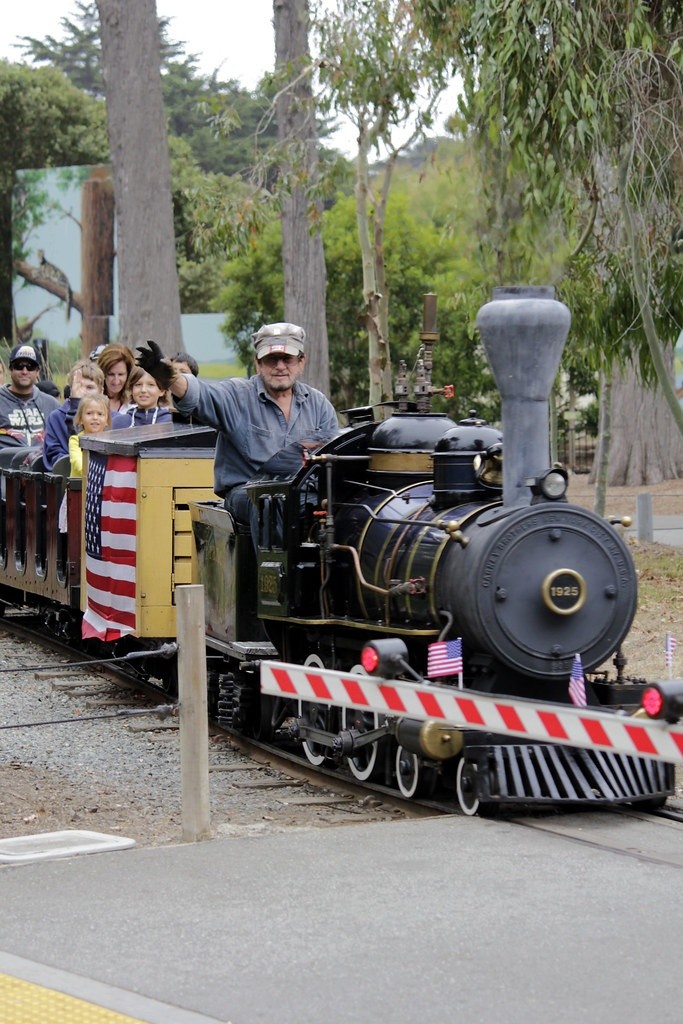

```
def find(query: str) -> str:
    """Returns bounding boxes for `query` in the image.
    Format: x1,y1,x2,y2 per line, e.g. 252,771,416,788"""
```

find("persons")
126,369,174,425
58,393,112,533
170,352,198,377
134,323,339,556
43,360,141,473
0,344,136,447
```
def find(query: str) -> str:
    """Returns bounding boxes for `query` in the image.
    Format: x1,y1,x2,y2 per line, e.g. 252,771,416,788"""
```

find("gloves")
135,339,179,390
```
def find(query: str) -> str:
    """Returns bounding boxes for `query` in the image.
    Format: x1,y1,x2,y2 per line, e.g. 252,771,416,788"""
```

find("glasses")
13,362,38,371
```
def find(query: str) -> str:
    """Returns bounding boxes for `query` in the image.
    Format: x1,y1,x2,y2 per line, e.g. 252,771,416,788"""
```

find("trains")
0,280,683,823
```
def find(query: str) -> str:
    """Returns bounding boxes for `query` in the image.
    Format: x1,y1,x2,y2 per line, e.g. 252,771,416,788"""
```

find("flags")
569,657,587,709
428,638,464,679
666,636,679,666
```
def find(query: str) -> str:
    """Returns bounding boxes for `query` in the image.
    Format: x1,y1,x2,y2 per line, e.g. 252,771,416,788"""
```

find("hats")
10,346,40,367
90,343,108,361
252,324,306,359
35,381,60,397
